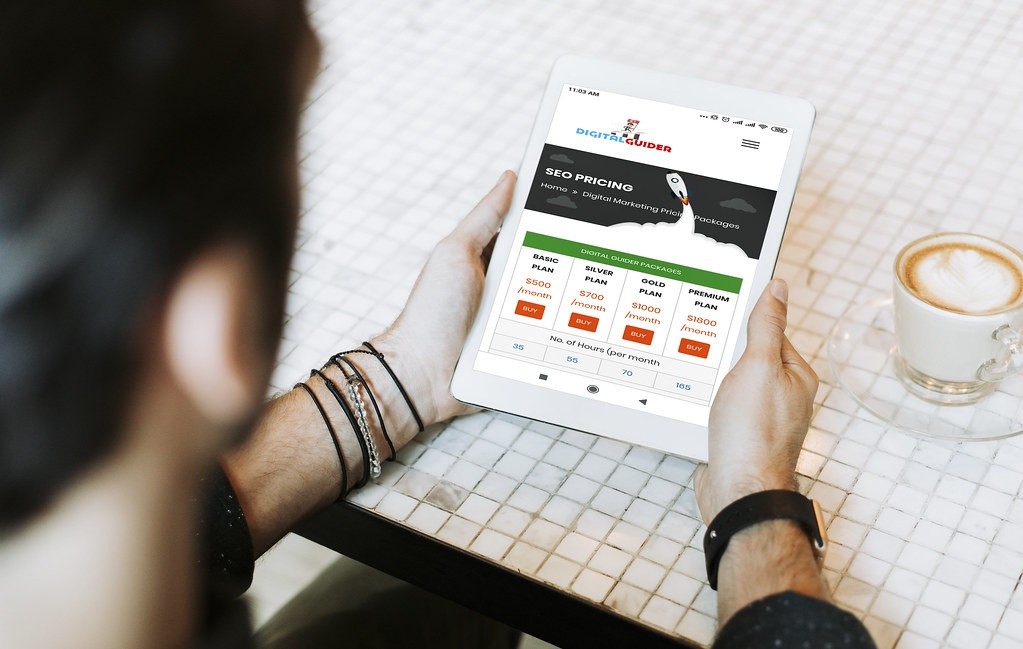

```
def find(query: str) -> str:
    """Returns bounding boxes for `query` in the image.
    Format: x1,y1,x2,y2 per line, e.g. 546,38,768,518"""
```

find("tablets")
449,55,817,465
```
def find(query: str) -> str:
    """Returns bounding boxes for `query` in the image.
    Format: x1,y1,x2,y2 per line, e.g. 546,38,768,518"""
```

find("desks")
268,0,1023,649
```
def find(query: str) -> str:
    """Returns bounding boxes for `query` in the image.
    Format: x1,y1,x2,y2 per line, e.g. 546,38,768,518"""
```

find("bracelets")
703,489,827,586
296,338,425,502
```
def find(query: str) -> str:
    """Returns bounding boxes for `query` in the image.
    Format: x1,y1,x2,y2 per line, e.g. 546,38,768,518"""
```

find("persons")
0,0,870,647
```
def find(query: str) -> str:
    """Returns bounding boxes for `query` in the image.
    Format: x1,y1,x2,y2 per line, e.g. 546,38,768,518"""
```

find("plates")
829,296,1023,443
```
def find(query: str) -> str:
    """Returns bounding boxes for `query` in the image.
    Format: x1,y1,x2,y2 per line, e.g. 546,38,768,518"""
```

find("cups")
893,230,1023,405
